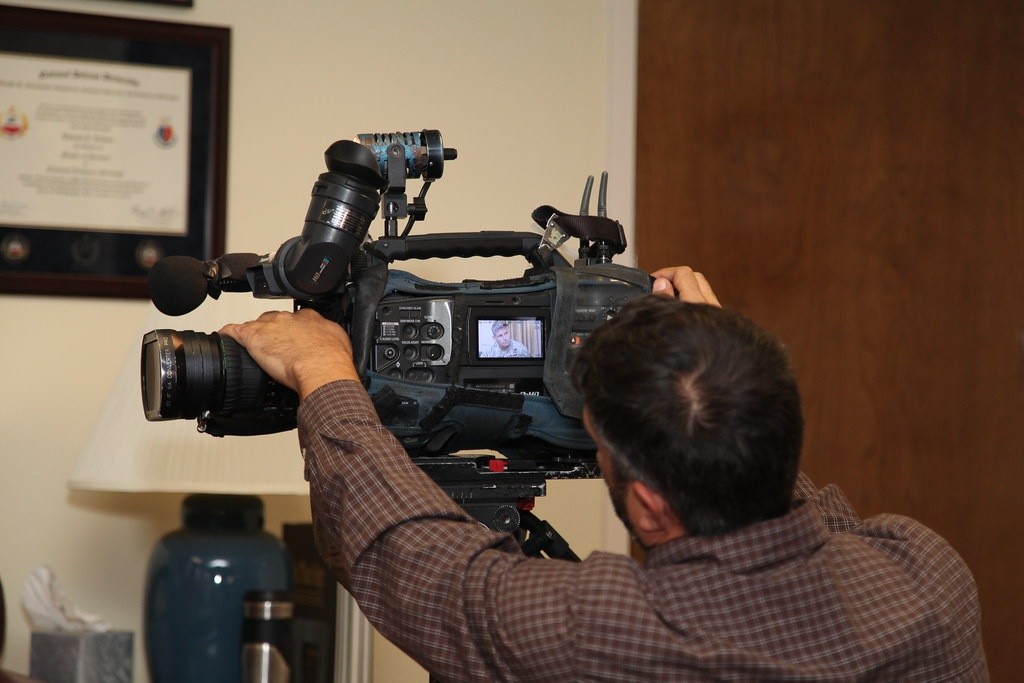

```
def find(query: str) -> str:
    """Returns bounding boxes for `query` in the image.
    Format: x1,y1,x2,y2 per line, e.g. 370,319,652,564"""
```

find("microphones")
149,254,262,316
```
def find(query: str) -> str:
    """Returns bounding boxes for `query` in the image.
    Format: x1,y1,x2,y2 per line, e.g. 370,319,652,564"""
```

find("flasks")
241,589,293,682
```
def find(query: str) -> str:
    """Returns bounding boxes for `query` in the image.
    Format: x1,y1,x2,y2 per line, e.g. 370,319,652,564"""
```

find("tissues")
20,565,134,683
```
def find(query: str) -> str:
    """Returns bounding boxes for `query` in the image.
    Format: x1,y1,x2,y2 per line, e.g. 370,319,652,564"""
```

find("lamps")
65,251,310,683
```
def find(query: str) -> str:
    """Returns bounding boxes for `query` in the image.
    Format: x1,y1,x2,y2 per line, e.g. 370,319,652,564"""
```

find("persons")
217,266,990,683
481,321,529,358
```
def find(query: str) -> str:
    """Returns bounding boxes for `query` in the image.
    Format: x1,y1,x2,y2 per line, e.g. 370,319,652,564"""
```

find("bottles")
145,493,286,683
